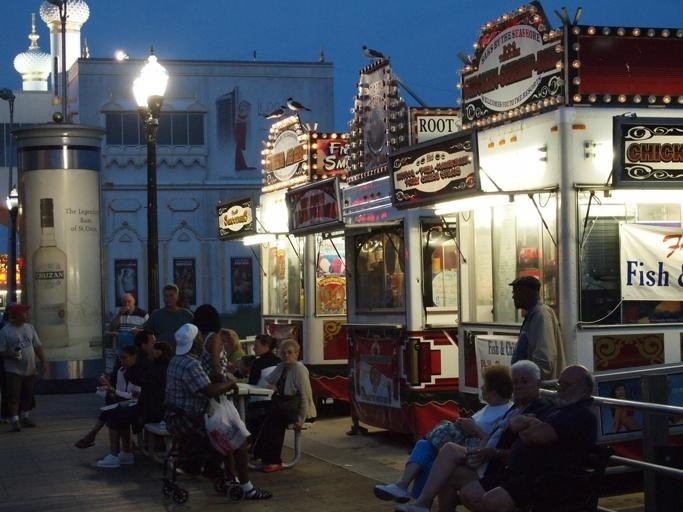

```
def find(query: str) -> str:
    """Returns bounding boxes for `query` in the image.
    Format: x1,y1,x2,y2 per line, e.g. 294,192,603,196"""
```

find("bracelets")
112,388,114,393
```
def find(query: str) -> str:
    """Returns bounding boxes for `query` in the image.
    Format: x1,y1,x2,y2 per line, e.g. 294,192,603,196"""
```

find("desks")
221,382,274,478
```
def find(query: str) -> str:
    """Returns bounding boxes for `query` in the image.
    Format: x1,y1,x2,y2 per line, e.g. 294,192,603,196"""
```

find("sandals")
75,438,96,449
242,487,272,500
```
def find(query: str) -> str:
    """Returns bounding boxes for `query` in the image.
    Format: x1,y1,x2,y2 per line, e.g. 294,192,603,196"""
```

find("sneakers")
262,464,281,472
119,451,135,464
11,420,21,432
394,503,429,512
21,417,35,427
96,453,120,468
374,483,411,504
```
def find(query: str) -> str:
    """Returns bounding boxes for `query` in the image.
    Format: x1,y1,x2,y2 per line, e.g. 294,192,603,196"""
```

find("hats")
174,323,198,355
508,276,541,289
7,304,30,313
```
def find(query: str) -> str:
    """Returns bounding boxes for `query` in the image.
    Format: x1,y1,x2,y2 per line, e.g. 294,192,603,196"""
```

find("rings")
477,448,481,453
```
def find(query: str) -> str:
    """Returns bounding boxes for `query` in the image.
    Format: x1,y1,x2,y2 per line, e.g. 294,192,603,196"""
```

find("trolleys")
158,376,246,502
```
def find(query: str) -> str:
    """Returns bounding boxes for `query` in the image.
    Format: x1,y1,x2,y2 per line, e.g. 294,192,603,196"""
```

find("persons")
239,355,256,383
129,284,195,344
250,339,317,473
246,334,283,385
234,100,258,171
508,276,566,381
163,323,273,500
0,302,15,330
394,359,552,511
203,332,229,382
116,269,135,300
374,363,514,504
459,364,599,511
107,292,150,353
611,384,644,432
74,345,138,449
0,304,49,432
94,342,173,468
217,329,245,374
177,268,191,309
135,329,156,355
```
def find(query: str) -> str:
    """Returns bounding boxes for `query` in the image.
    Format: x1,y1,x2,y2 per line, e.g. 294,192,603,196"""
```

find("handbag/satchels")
271,369,301,417
427,419,464,449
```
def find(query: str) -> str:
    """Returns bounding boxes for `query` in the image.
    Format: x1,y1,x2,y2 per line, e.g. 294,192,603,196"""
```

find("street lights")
5,185,20,312
5,185,18,304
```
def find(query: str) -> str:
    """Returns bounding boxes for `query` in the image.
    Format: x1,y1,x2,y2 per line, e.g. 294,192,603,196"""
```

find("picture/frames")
114,257,138,306
231,257,253,304
173,257,193,304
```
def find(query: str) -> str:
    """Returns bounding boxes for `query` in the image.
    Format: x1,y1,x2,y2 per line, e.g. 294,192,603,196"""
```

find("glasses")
553,383,584,391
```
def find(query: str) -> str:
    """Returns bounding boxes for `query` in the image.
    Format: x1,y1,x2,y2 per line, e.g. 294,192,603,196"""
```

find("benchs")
131,419,176,468
238,396,311,471
569,441,616,512
